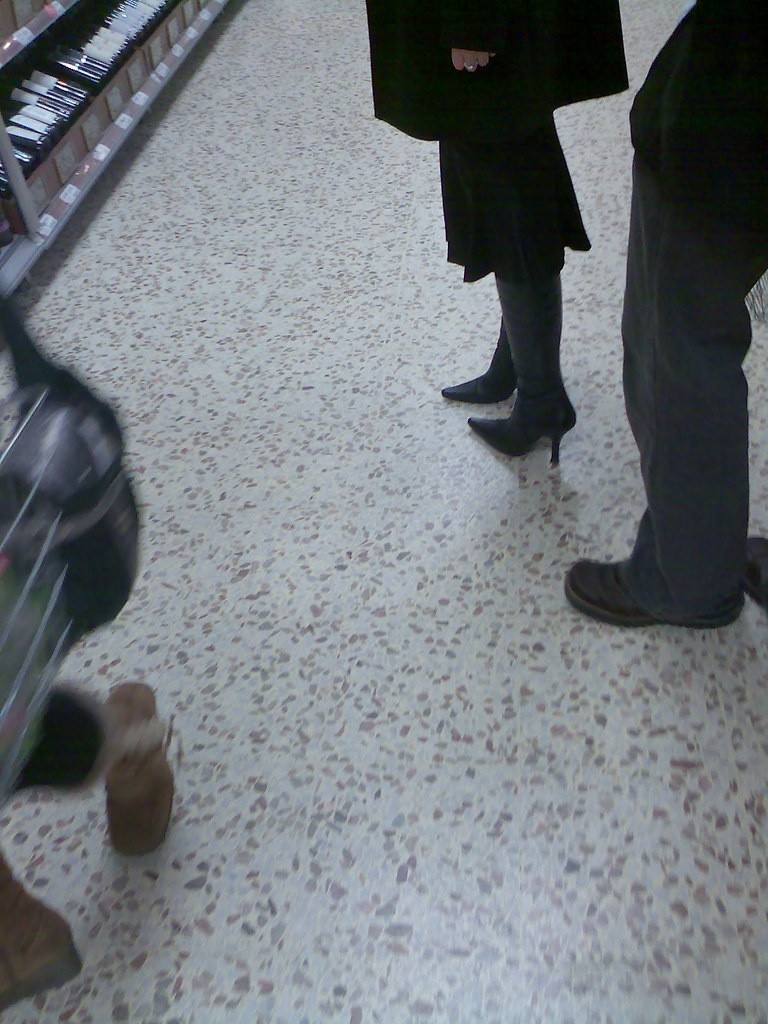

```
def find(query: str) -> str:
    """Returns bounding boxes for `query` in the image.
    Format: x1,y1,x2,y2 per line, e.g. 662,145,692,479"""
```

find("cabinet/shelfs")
0,1,229,303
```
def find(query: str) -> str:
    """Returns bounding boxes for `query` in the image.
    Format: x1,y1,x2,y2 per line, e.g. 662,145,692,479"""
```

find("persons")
565,0,768,632
0,283,176,1007
366,0,630,467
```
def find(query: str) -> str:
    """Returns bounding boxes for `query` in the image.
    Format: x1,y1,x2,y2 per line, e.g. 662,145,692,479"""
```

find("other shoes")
741,537,768,612
564,557,744,629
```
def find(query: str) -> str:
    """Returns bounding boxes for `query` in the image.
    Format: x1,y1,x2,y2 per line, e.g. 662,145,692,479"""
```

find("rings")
464,60,479,71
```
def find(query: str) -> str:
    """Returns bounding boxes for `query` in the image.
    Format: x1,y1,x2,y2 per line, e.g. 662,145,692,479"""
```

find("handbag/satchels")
0,368,140,639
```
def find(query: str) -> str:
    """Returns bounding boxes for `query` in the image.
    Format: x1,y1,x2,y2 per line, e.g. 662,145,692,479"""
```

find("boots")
468,270,577,464
0,854,81,1011
441,313,522,404
86,681,174,855
